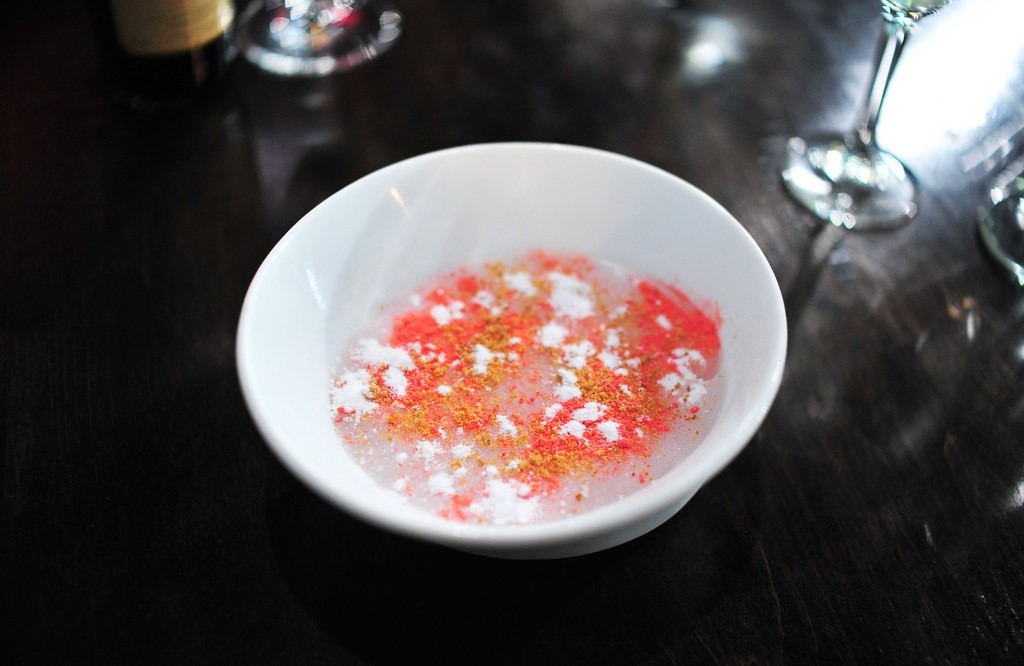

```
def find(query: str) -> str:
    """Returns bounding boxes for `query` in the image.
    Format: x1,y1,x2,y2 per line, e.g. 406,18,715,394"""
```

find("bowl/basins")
235,140,788,569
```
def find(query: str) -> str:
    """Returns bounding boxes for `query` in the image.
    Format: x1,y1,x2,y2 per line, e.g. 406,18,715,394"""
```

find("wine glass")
779,0,947,232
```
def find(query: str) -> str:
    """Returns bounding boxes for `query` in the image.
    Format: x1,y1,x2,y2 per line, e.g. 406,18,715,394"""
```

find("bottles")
110,0,236,70
